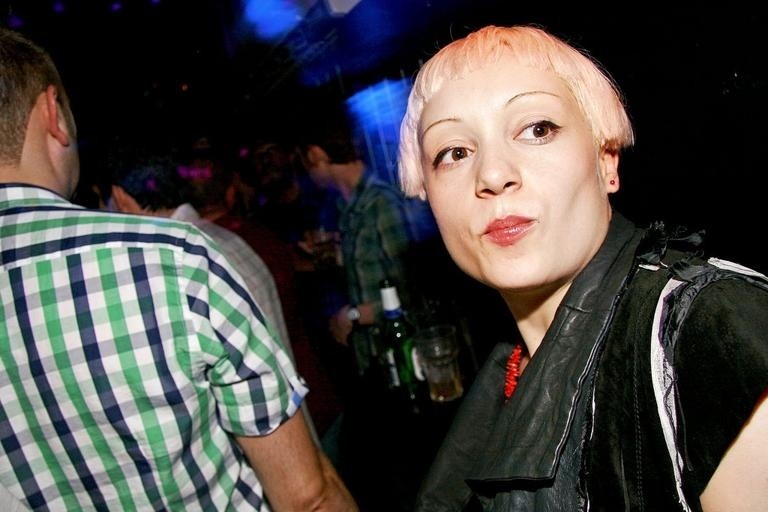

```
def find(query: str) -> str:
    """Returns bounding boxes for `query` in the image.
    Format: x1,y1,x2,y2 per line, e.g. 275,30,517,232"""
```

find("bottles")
379,279,426,413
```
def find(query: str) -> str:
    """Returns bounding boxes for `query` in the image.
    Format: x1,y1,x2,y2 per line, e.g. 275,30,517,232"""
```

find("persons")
92,111,417,419
396,25,768,510
1,28,356,512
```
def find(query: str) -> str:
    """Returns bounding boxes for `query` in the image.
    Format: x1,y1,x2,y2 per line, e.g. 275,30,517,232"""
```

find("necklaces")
503,346,522,397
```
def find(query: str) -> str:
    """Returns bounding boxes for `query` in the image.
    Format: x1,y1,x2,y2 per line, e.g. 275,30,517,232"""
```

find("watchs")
348,304,361,327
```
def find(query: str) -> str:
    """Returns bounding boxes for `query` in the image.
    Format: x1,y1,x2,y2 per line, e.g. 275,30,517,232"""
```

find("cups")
422,325,464,402
